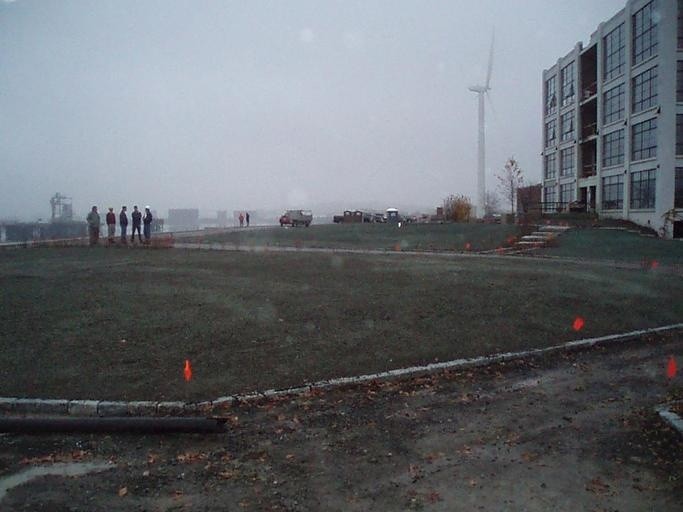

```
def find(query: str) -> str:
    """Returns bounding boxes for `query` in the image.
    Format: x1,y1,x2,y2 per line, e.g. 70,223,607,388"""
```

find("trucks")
279,209,312,227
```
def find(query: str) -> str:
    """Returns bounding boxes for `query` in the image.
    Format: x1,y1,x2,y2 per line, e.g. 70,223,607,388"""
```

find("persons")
131,206,142,243
142,205,153,245
107,208,117,245
87,205,101,249
239,214,244,226
245,212,250,228
118,205,129,246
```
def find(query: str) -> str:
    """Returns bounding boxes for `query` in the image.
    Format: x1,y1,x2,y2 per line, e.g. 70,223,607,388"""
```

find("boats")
4,193,87,239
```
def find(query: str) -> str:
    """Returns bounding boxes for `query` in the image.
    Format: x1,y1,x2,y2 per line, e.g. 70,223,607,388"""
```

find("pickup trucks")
332,208,417,225
